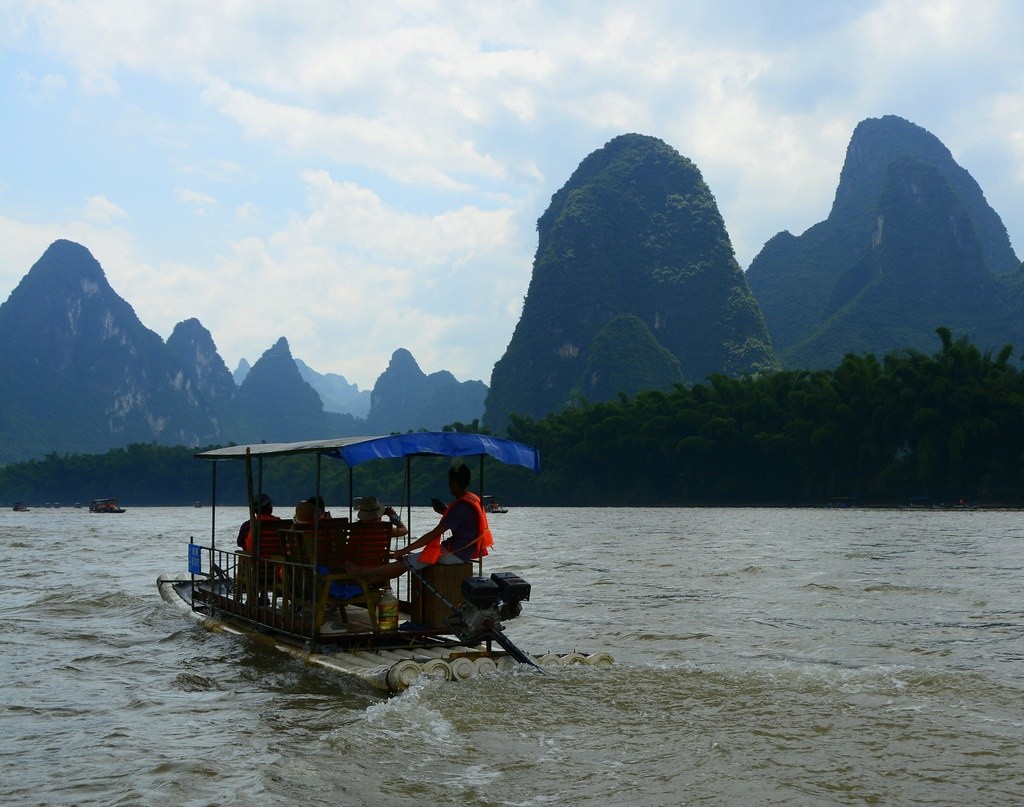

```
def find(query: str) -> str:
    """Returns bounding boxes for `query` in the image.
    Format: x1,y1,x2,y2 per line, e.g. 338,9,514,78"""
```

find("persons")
354,461,494,583
285,502,334,620
96,503,117,509
299,495,329,523
350,495,408,566
236,493,284,606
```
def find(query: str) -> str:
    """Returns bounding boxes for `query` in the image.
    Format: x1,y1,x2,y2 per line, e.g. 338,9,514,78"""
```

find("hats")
293,502,322,523
357,496,385,520
306,497,325,514
253,495,275,508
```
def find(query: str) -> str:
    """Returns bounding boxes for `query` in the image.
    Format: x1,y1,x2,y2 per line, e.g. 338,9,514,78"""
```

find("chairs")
274,529,377,633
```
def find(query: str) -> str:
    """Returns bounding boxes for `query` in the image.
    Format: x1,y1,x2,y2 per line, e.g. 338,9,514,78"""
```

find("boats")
157,432,616,692
482,495,509,515
352,496,363,509
88,498,127,513
73,503,82,508
13,502,31,511
45,503,62,508
192,501,202,507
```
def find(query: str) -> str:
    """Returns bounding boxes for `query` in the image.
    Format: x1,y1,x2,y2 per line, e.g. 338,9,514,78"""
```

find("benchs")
233,550,274,604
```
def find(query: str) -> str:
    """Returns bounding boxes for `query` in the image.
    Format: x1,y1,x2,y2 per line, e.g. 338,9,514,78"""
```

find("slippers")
396,620,410,630
399,622,428,631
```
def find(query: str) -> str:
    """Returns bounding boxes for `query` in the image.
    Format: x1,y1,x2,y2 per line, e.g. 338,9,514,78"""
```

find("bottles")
378,587,398,630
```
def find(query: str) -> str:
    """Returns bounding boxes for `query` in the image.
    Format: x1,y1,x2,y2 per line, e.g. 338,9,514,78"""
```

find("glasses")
271,503,274,507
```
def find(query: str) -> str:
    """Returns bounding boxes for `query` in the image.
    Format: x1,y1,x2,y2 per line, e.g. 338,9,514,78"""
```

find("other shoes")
258,597,270,606
319,621,349,634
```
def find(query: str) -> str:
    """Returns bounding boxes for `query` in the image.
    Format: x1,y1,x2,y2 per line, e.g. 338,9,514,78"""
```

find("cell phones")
432,499,444,511
